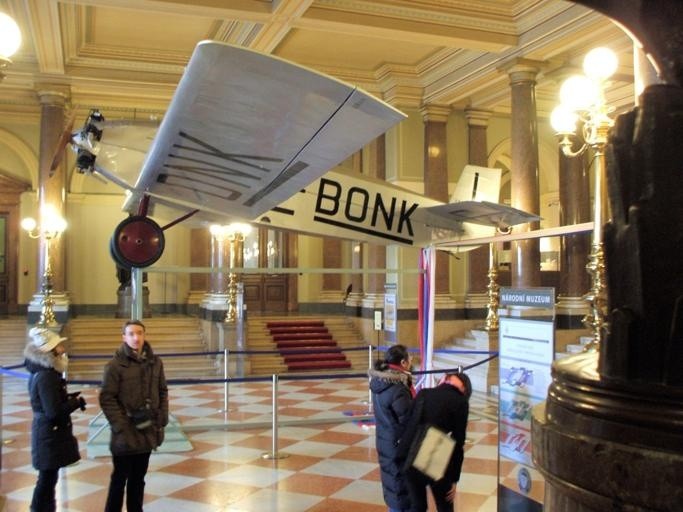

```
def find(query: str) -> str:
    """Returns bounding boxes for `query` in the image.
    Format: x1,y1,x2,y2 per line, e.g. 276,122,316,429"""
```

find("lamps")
0,11,21,83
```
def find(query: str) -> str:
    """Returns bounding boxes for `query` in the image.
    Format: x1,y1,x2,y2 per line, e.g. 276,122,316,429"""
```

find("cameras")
67,392,86,414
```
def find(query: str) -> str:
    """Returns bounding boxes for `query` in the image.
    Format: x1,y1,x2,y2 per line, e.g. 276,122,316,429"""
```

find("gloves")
67,391,86,411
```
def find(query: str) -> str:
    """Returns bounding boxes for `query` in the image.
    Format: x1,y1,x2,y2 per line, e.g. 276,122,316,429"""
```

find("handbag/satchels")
401,424,457,482
110,406,158,454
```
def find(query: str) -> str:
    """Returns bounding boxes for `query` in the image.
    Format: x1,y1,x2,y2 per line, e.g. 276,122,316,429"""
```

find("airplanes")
48,39,545,269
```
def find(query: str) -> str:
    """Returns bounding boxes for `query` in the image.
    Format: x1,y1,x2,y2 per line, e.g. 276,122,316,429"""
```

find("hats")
28,327,68,354
447,371,472,401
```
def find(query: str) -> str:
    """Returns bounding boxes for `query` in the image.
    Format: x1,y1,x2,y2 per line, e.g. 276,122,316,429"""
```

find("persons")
98,320,170,511
391,372,472,511
366,344,429,511
23,327,85,511
